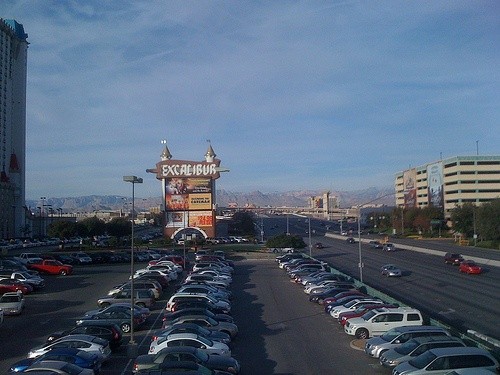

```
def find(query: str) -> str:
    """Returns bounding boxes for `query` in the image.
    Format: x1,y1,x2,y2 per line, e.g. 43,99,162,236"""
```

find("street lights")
37,206,42,236
21,205,27,235
12,205,15,238
49,205,63,232
121,175,144,343
358,186,415,283
40,196,46,240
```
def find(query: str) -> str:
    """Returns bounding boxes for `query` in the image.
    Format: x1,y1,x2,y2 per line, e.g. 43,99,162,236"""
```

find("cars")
443,252,465,266
205,235,250,244
315,243,323,249
378,264,403,278
268,246,500,375
346,237,355,244
298,217,386,235
120,230,164,243
381,243,396,251
284,232,290,236
0,234,114,248
0,248,241,375
458,260,483,274
368,240,383,249
